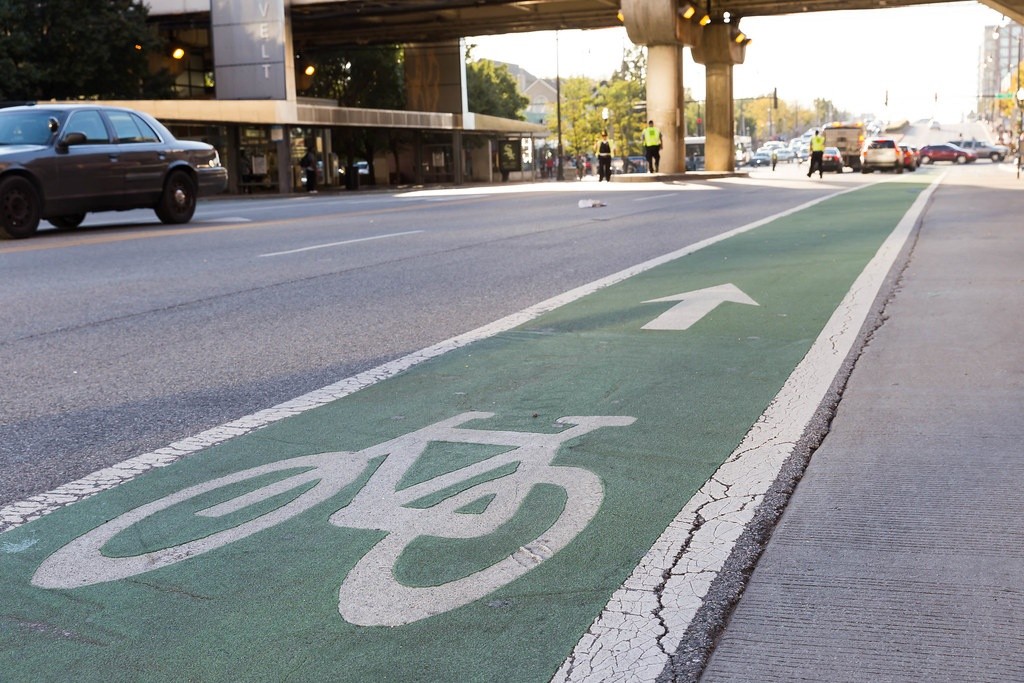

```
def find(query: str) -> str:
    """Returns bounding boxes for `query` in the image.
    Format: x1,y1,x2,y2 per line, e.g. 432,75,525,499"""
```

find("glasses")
602,135,607,137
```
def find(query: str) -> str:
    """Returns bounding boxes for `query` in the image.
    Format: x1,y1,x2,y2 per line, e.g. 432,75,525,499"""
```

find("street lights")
1017,87,1024,134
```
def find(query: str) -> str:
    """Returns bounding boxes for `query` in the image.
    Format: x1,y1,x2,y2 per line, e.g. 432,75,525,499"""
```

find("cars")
584,155,648,174
750,132,814,167
356,161,370,175
1,103,229,241
898,145,920,172
918,143,978,164
822,147,844,174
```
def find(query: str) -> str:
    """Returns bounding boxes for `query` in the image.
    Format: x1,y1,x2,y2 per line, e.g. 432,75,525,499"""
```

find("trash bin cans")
293,165,303,193
389,173,404,184
345,166,360,190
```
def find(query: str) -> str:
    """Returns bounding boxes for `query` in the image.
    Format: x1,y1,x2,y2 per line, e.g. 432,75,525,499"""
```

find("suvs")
947,140,1008,163
860,137,903,174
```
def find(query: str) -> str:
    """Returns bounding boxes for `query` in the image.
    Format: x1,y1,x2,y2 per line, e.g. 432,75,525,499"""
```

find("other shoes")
650,168,653,173
807,173,811,177
656,169,658,172
606,177,609,181
819,172,822,178
599,178,602,181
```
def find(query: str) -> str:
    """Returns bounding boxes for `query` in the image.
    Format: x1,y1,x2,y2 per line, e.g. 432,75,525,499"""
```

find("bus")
684,135,753,167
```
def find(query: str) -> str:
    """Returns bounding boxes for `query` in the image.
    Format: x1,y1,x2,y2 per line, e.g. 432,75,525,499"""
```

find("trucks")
822,122,864,172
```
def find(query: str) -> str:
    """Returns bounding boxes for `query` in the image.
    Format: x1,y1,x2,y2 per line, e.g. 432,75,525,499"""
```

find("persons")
772,152,777,171
305,147,318,193
595,135,614,182
642,120,663,173
241,156,250,192
546,157,553,178
807,130,825,178
